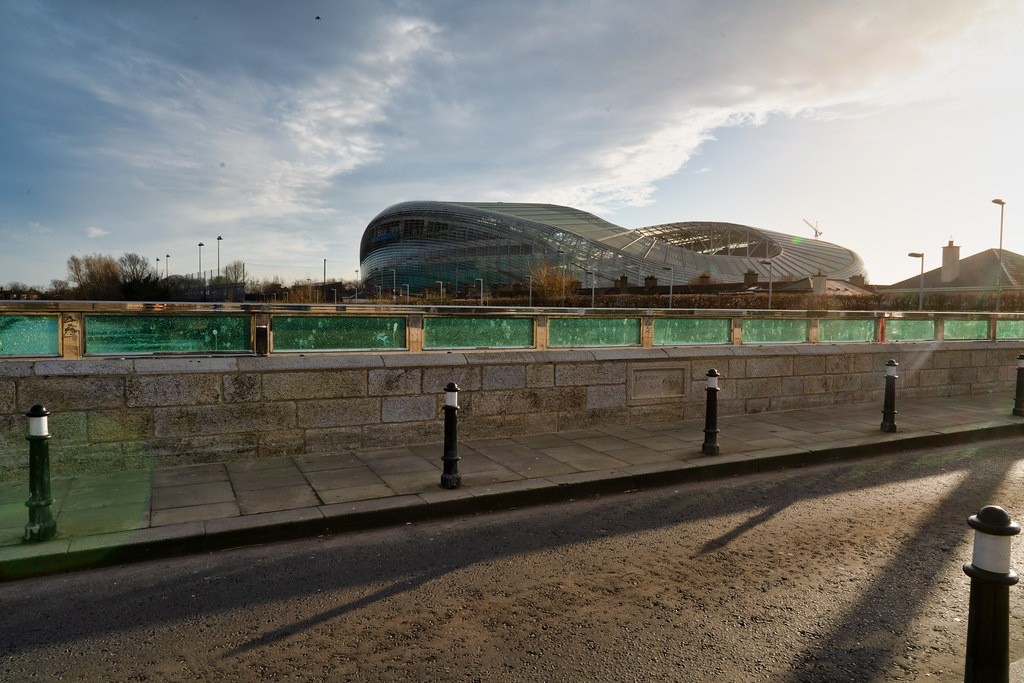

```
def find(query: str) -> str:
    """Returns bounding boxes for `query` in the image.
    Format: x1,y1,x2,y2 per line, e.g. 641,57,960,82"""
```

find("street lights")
475,278,484,304
388,266,396,303
761,260,773,309
272,292,278,304
197,242,204,280
306,278,313,302
216,235,223,277
281,291,290,303
909,252,926,312
990,199,1007,313
663,267,675,308
331,288,338,304
525,274,533,306
165,254,171,279
156,257,160,277
436,281,444,303
353,287,358,304
354,268,360,289
373,284,382,300
585,269,594,309
402,284,410,306
